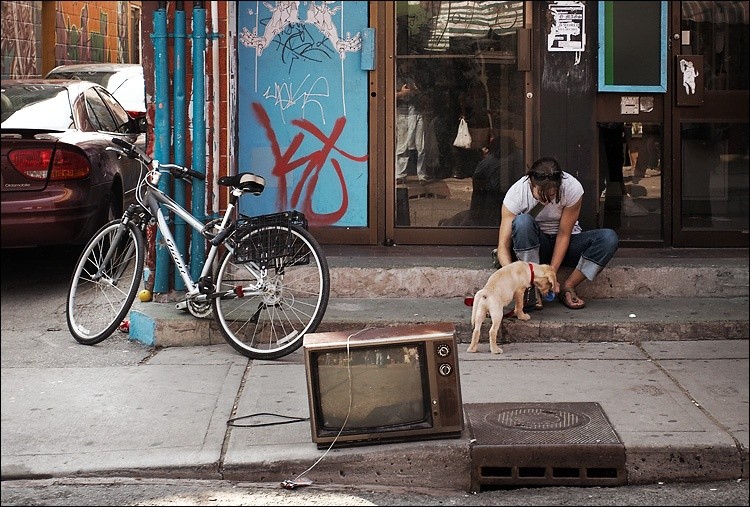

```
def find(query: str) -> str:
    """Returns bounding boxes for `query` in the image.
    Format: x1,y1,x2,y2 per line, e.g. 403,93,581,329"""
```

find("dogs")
467,261,560,354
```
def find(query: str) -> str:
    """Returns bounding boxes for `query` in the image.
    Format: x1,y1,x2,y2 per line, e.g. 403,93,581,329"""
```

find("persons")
394,46,525,227
494,157,619,310
597,51,750,225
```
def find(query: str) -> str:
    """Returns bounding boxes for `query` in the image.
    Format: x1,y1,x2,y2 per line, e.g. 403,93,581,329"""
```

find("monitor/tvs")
303,322,465,449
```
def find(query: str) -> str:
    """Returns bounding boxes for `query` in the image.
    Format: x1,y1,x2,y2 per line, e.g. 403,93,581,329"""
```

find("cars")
1,79,148,281
43,64,147,119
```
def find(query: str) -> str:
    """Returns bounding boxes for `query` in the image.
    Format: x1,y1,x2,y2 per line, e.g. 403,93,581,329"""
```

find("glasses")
530,171,562,182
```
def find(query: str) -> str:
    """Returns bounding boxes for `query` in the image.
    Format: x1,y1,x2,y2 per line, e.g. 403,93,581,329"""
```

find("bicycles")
66,139,330,360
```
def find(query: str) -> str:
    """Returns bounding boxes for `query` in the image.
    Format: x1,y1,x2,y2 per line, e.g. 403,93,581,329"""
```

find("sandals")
527,289,544,309
558,287,585,309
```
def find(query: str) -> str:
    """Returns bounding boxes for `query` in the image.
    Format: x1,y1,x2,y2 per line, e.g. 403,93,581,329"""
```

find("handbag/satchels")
453,119,472,149
492,240,517,270
